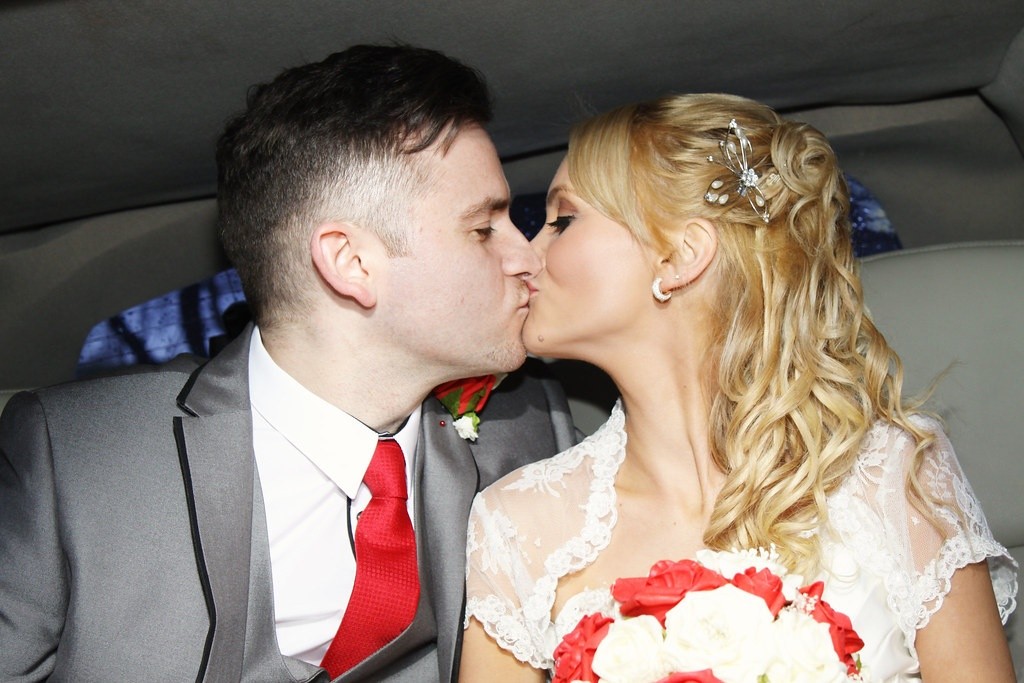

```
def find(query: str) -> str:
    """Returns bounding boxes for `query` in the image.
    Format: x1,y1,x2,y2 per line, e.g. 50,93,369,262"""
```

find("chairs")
861,241,1024,682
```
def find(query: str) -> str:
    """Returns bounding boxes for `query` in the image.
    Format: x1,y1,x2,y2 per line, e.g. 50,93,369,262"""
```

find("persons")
0,42,573,683
457,91,1019,683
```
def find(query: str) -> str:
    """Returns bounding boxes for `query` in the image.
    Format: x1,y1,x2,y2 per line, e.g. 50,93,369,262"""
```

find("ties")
319,441,419,681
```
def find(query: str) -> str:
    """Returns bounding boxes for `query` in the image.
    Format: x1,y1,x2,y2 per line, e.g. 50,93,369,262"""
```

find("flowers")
546,547,860,683
432,374,503,442
